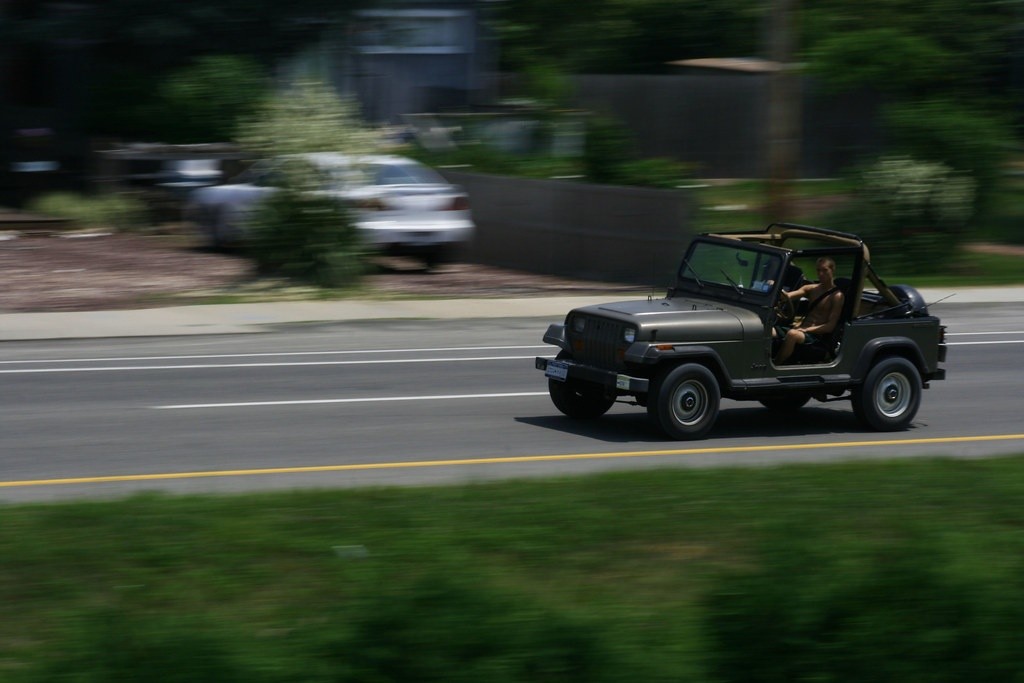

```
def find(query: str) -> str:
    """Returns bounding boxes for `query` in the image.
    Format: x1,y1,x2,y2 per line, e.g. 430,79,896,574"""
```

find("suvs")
534,219,950,440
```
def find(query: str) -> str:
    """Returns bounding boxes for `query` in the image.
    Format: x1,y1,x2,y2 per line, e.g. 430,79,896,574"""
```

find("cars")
179,147,478,266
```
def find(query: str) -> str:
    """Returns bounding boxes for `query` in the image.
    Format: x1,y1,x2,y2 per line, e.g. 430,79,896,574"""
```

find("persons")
766,255,845,365
